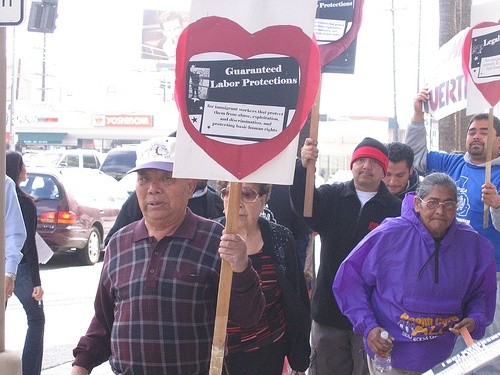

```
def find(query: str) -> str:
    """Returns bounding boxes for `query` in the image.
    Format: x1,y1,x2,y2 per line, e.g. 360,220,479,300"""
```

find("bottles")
373,331,393,372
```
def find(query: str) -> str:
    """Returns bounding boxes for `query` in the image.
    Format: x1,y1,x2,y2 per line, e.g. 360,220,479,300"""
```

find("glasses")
220,188,261,204
419,197,456,210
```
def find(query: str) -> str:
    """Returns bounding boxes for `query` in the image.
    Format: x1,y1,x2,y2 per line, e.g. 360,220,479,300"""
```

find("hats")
350,137,388,177
125,137,177,174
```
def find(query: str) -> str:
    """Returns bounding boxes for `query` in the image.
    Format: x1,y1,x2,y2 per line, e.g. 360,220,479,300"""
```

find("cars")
21,148,107,170
99,142,160,196
8,165,132,267
